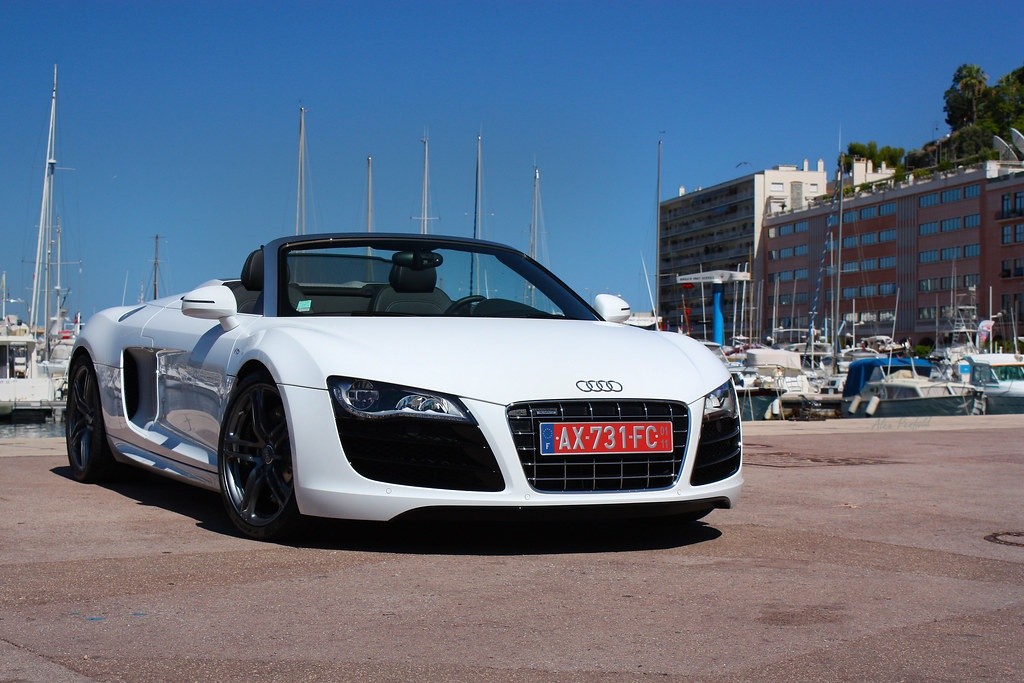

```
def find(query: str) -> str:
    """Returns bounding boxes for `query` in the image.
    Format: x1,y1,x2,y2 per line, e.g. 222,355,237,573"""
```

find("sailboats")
1,60,1024,426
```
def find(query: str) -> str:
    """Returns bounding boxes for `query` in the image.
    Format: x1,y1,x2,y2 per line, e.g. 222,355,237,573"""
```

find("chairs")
223,248,312,316
374,264,454,315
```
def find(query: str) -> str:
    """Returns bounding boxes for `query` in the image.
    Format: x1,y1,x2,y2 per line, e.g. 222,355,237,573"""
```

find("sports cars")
67,232,746,544
